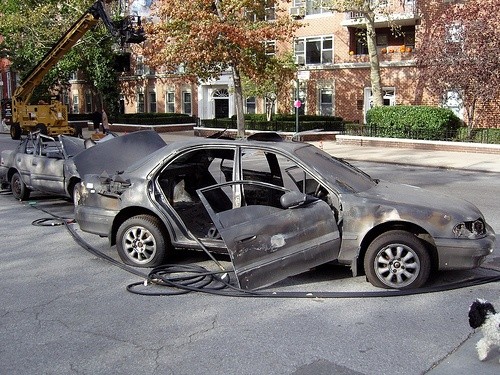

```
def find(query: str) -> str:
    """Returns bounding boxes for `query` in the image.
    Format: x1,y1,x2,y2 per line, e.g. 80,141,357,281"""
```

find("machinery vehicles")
0,0,148,140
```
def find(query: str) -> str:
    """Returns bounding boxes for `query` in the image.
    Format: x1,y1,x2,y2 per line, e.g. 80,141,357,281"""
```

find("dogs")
467,297,500,365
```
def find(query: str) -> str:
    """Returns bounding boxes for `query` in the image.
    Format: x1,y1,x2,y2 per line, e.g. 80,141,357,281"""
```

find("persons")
101,109,109,135
4,104,12,116
92,108,103,134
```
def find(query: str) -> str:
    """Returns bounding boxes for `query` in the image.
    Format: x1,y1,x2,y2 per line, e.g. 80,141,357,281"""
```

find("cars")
0,130,119,201
72,129,495,290
